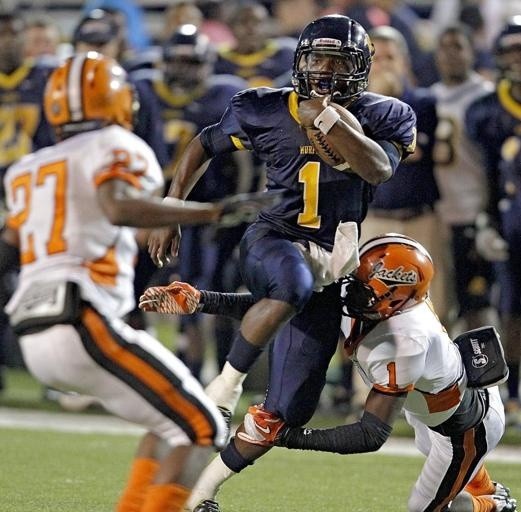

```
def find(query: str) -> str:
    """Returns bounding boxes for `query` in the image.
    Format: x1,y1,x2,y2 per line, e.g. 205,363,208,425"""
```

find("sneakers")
203,375,243,452
478,480,516,512
184,495,221,512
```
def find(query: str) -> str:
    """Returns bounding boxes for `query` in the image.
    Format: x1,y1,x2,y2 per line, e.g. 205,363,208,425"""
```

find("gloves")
139,281,200,315
238,403,285,446
218,190,281,227
475,227,509,261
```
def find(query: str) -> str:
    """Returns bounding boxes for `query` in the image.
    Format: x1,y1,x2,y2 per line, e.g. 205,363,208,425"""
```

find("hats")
73,11,116,44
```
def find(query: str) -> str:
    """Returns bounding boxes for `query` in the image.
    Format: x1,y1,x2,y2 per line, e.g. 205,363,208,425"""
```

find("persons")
148,14,417,511
0,52,271,511
140,232,516,512
1,1,521,425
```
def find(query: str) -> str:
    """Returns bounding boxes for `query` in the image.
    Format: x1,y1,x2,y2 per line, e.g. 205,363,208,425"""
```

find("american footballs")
306,101,363,174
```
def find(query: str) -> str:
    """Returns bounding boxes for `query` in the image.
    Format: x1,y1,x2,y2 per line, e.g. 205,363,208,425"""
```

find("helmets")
336,233,434,320
293,15,372,101
495,24,520,83
163,27,211,95
44,54,134,126
